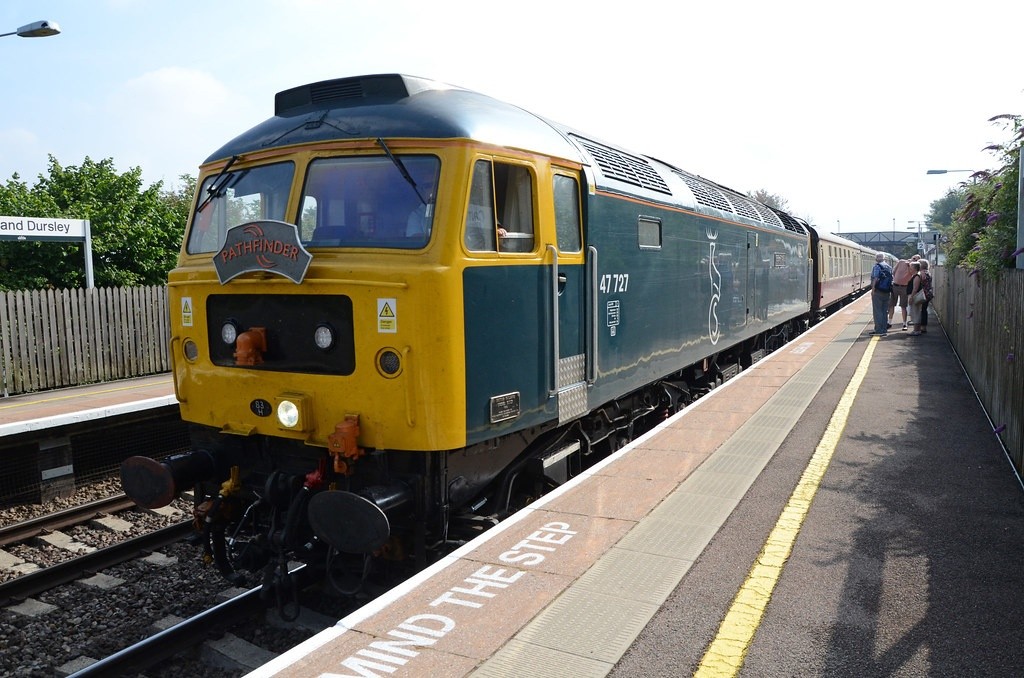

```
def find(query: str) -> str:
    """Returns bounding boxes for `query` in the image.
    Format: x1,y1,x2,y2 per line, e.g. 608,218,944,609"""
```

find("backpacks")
876,263,892,293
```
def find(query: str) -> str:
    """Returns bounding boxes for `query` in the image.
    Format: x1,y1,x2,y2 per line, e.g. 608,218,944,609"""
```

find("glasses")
424,182,433,189
913,257,917,261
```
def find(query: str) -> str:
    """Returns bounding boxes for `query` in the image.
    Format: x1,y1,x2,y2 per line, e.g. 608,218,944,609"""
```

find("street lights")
893,218,895,249
907,221,922,259
926,169,976,185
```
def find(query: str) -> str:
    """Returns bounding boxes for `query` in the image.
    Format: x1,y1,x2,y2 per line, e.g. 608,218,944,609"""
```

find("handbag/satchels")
913,274,927,305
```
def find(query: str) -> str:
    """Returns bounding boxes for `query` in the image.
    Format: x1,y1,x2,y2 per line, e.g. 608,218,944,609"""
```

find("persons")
869,253,893,336
887,254,933,337
406,174,508,254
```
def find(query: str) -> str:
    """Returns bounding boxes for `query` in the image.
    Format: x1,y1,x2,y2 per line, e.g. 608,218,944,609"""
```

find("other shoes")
908,321,914,325
902,324,908,330
917,331,922,335
869,332,883,336
907,330,918,336
888,323,893,328
921,329,927,333
883,332,887,336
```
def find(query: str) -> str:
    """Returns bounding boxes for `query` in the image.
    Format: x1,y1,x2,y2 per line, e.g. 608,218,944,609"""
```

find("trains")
120,73,900,623
